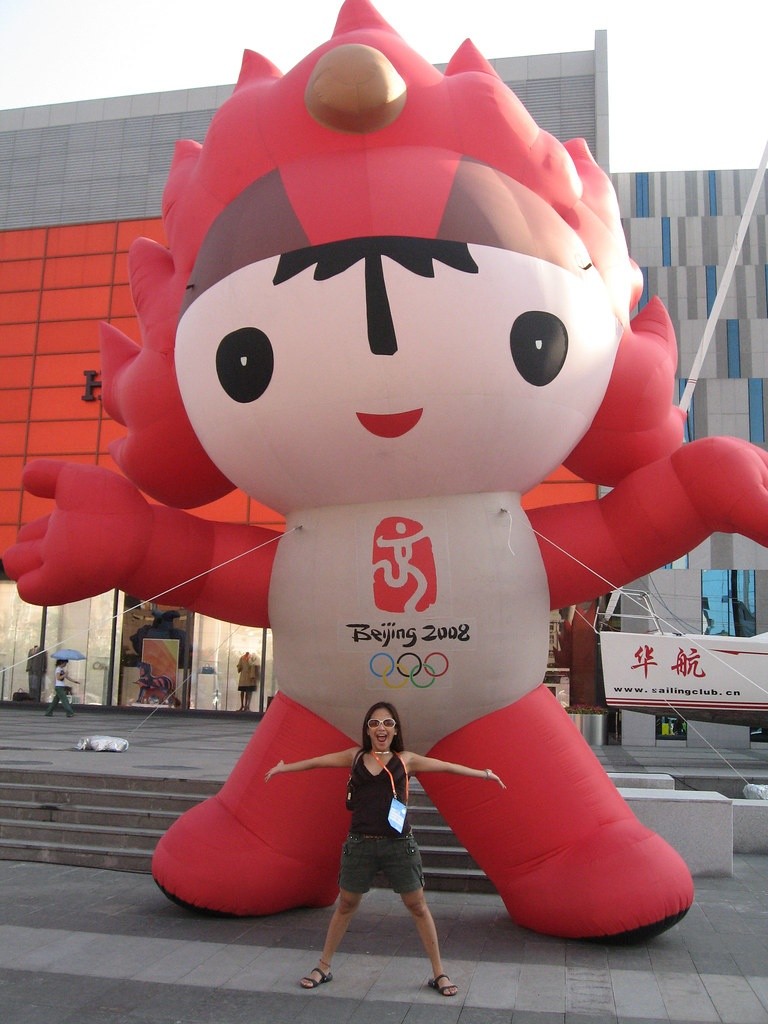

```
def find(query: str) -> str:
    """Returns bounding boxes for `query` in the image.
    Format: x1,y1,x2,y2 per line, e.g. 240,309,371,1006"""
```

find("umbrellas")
50,649,86,670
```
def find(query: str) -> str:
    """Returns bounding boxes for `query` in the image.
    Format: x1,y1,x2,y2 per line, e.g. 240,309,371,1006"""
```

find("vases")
568,713,609,745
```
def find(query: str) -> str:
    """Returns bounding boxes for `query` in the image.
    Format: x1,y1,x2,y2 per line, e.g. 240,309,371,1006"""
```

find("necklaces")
374,751,390,755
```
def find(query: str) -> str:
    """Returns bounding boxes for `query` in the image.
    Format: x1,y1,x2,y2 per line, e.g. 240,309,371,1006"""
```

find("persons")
236,652,257,711
264,702,507,996
25,645,47,702
45,659,80,717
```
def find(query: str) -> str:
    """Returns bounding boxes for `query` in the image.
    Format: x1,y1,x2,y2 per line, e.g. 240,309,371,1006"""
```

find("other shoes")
67,715,73,717
45,714,52,716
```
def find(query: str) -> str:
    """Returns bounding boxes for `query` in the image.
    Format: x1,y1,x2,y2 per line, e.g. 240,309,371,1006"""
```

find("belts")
349,827,412,839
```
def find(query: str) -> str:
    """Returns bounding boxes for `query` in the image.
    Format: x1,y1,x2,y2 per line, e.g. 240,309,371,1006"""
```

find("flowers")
564,703,609,715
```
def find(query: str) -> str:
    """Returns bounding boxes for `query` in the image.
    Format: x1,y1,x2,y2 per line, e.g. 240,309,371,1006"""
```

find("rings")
269,774,270,775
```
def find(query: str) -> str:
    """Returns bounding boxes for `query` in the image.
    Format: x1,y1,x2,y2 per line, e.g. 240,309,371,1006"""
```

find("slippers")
428,974,458,996
301,968,332,988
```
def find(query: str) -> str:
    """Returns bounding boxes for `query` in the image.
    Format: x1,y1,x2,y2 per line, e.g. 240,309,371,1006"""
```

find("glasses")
368,718,396,729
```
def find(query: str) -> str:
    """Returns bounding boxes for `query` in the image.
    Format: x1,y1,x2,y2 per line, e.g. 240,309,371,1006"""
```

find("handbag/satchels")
346,751,364,810
202,663,212,674
13,688,29,702
